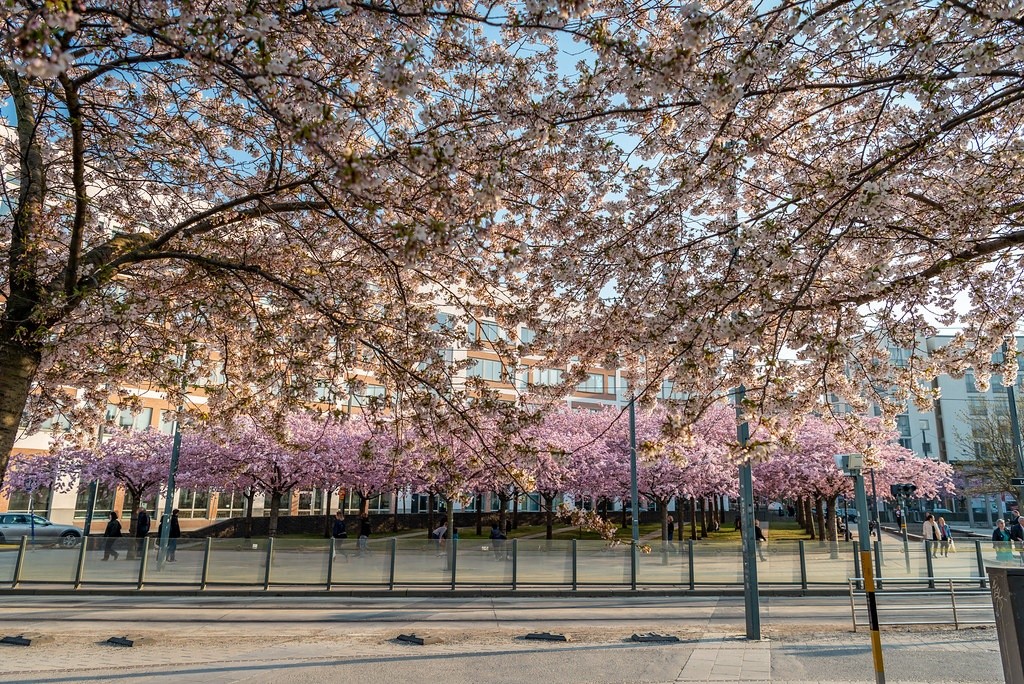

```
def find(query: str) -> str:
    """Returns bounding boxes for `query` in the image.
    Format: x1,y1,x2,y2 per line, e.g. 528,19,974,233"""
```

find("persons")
101,512,122,560
431,512,458,558
992,510,1024,562
358,513,370,558
921,511,953,559
668,516,678,554
778,505,795,518
837,517,853,540
155,509,181,564
332,509,348,563
488,524,507,562
755,520,767,562
136,507,151,557
894,505,902,533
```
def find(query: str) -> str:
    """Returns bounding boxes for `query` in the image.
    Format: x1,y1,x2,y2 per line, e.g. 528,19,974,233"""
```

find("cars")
0,513,85,548
